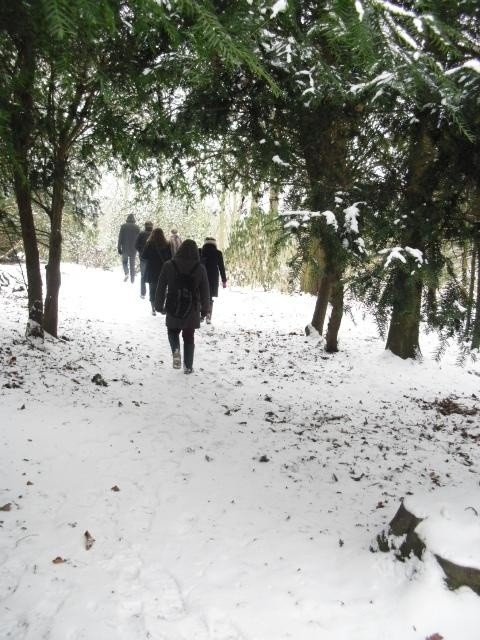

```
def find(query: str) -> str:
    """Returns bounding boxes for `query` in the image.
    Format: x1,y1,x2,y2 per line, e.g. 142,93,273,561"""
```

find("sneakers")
173,349,181,369
184,367,194,374
138,294,145,299
206,317,212,324
123,273,129,282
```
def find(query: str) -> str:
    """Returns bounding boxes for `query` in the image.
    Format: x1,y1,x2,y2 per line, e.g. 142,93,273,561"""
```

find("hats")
203,237,217,247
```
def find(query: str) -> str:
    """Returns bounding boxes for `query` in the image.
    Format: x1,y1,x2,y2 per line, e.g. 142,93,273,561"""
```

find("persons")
117,214,141,283
196,236,229,325
134,221,155,298
141,228,171,316
154,238,211,375
167,229,184,256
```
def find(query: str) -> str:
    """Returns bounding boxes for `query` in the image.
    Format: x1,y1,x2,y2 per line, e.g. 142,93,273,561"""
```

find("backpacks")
163,258,201,320
